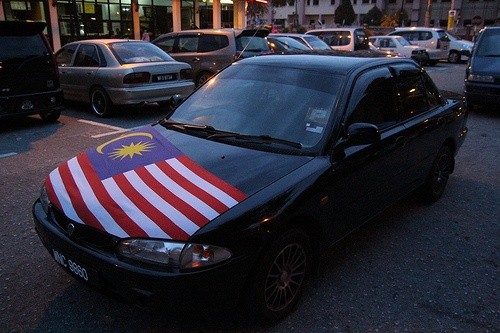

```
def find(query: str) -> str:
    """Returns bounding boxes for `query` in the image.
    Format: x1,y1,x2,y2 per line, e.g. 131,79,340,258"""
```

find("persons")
250,12,260,25
142,27,159,42
191,23,198,29
108,26,133,39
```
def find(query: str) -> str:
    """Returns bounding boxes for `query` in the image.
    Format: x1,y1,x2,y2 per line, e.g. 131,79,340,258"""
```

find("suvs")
0,19,500,122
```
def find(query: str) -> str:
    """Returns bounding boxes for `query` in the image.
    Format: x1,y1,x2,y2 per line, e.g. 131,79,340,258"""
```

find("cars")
31,54,469,323
